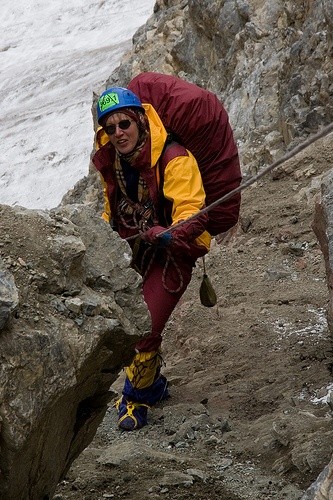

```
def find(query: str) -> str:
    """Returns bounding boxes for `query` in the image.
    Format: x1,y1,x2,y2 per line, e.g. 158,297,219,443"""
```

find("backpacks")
126,71,243,237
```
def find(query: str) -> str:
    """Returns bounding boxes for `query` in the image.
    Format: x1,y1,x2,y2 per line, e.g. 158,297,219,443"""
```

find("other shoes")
113,346,169,431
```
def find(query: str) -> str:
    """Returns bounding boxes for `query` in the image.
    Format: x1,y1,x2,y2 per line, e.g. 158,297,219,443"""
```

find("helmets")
96,86,145,126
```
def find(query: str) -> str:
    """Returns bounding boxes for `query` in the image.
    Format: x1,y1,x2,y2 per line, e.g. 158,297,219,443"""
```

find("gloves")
142,226,171,241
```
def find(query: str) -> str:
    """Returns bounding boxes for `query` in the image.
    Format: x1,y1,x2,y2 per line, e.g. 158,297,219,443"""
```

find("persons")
91,86,211,429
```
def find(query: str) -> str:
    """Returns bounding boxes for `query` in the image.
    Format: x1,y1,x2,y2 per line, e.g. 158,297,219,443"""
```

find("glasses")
105,119,135,136
